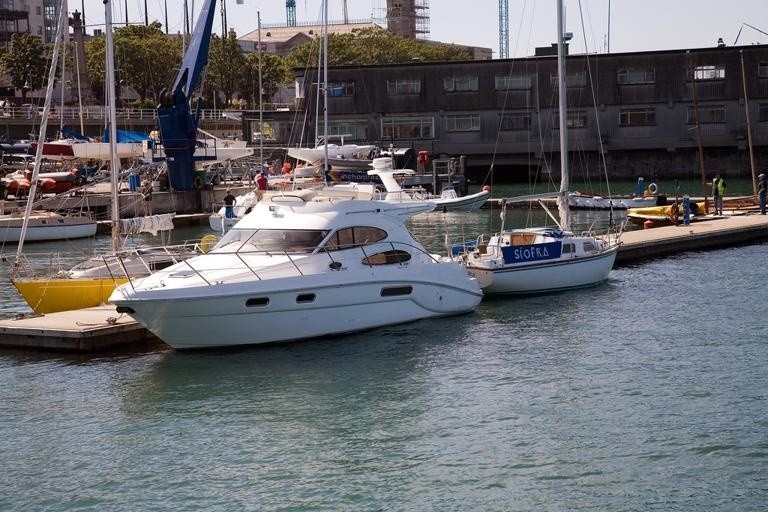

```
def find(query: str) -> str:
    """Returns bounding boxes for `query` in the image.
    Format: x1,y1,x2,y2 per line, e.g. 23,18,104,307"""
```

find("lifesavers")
198,235,217,253
649,184,658,194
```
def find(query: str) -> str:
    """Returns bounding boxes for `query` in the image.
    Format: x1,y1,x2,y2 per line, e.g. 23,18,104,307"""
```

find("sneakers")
713,212,722,216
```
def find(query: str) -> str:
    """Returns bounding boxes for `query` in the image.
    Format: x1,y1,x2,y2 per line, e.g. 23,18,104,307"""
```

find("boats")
624,200,749,228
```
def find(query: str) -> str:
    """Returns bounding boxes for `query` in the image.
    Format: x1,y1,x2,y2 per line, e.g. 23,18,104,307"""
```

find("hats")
757,174,765,178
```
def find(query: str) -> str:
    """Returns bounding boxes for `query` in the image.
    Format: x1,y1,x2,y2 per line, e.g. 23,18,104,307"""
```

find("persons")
257,173,268,189
223,189,236,218
312,163,343,184
254,169,266,188
757,173,767,215
712,174,727,216
